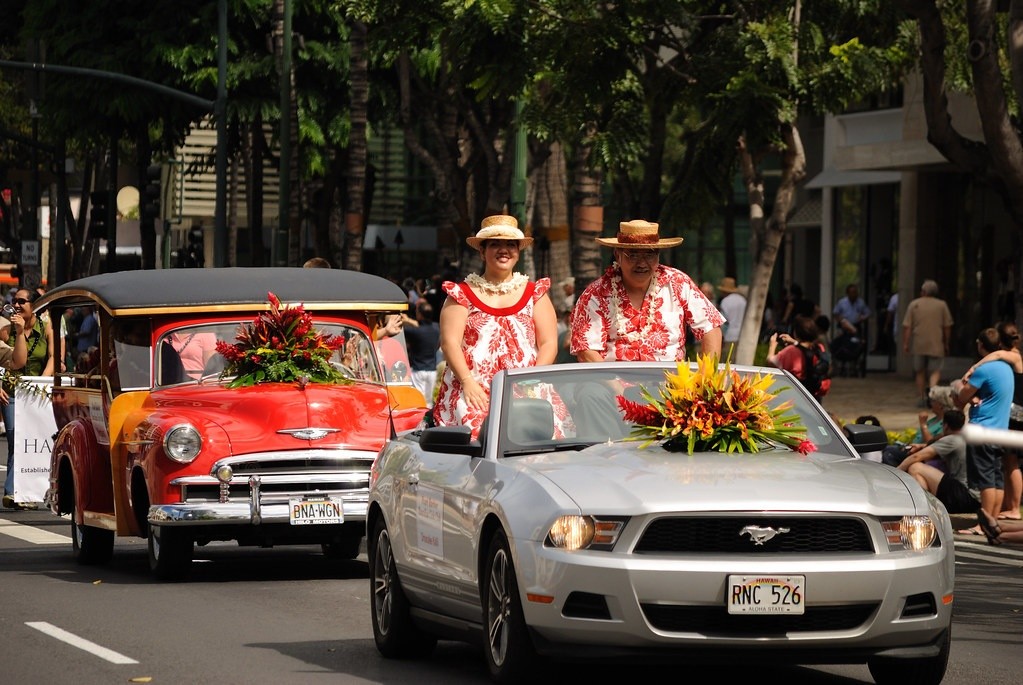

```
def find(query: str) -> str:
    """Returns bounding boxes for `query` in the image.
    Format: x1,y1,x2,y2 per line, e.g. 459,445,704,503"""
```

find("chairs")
472,399,553,444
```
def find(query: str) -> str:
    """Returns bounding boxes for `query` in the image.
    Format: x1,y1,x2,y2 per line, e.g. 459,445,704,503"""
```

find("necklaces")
13,315,43,358
166,329,199,354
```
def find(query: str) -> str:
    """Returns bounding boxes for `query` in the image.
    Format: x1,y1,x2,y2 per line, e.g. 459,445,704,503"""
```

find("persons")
558,276,576,314
697,274,898,405
569,220,726,437
882,320,1023,546
903,279,954,408
374,268,456,415
0,285,220,512
433,215,578,449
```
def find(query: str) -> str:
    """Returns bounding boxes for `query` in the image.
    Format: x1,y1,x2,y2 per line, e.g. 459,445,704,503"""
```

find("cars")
29,266,434,579
366,363,956,685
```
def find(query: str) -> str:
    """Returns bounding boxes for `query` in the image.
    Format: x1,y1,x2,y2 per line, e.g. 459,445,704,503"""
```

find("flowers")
215,291,357,389
615,342,818,456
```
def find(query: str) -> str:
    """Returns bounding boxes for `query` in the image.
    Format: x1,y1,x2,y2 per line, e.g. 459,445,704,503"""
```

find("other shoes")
0,465,7,471
0,426,6,437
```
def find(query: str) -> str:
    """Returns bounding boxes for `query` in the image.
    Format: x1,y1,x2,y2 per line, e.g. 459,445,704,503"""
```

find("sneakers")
2,495,26,510
24,502,38,510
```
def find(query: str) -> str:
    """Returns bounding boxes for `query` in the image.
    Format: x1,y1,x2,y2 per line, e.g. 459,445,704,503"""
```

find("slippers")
958,528,985,536
998,512,1022,520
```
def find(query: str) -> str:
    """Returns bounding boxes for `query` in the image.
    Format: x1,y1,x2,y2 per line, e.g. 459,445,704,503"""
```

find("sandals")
977,508,1002,546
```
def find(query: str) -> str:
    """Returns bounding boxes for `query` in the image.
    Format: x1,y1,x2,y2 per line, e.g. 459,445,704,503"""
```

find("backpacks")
794,341,831,398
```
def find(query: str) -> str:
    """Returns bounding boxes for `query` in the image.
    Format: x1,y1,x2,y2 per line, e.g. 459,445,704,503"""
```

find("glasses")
12,297,30,304
622,249,660,261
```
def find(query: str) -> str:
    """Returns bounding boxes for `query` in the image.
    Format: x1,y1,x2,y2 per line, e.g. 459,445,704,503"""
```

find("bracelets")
921,425,927,430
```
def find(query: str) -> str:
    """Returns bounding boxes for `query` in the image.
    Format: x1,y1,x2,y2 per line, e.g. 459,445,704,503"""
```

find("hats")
466,215,534,251
595,220,683,248
717,278,738,293
558,277,575,287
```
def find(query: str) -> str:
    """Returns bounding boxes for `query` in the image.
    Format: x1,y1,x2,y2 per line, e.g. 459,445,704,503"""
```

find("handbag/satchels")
2,356,30,397
